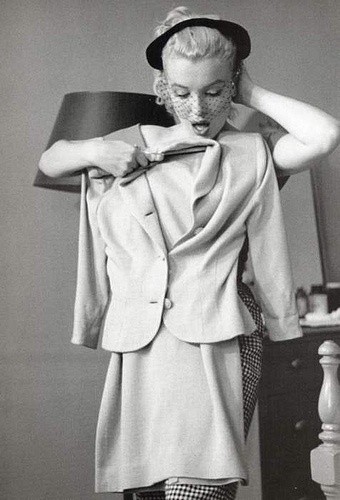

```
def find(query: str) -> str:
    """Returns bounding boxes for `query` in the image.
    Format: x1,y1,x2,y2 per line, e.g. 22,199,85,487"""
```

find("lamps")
31,90,175,189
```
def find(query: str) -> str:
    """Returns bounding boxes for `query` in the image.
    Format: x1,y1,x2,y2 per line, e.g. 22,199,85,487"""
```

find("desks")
256,322,340,500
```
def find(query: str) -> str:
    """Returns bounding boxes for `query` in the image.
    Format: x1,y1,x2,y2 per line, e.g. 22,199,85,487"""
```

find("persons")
37,4,340,500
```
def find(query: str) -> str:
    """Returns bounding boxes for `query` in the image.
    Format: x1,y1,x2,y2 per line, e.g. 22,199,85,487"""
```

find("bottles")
296,287,310,318
308,286,328,314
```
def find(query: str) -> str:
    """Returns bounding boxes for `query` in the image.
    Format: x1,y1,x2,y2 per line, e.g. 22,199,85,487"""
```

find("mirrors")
274,165,329,300
